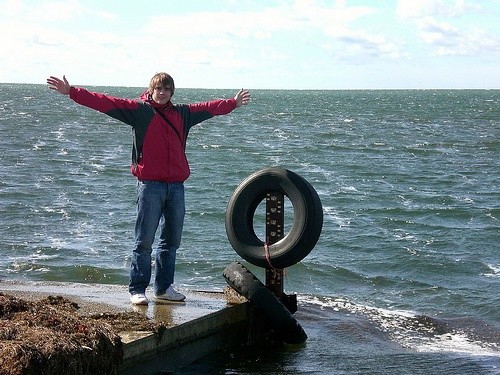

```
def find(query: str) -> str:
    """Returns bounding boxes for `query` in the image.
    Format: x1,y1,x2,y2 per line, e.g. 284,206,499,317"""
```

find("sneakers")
155,286,186,301
130,291,148,305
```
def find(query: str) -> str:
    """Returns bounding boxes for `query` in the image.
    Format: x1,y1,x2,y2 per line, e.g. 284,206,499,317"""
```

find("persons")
46,72,251,305
